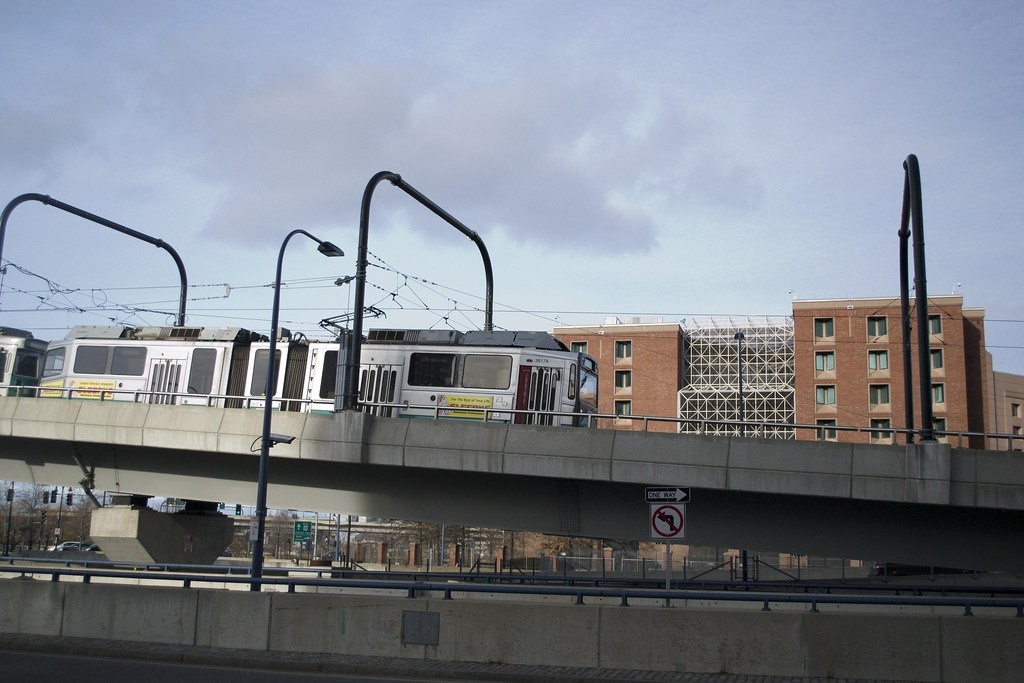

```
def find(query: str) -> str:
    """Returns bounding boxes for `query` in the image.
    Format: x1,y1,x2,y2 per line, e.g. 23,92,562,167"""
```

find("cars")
47,542,102,551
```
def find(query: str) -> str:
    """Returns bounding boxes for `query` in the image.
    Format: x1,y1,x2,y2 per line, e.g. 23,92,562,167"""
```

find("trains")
0,325,599,428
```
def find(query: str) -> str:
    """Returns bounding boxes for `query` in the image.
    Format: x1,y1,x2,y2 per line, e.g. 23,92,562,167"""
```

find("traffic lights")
42,490,57,504
66,493,73,505
41,511,47,525
7,489,14,501
236,504,241,515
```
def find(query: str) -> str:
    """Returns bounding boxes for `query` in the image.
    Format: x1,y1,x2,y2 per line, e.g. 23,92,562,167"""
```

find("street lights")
734,332,744,437
250,229,345,592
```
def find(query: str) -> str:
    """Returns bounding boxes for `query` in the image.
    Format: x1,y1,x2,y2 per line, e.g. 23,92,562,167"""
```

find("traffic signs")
293,520,312,542
